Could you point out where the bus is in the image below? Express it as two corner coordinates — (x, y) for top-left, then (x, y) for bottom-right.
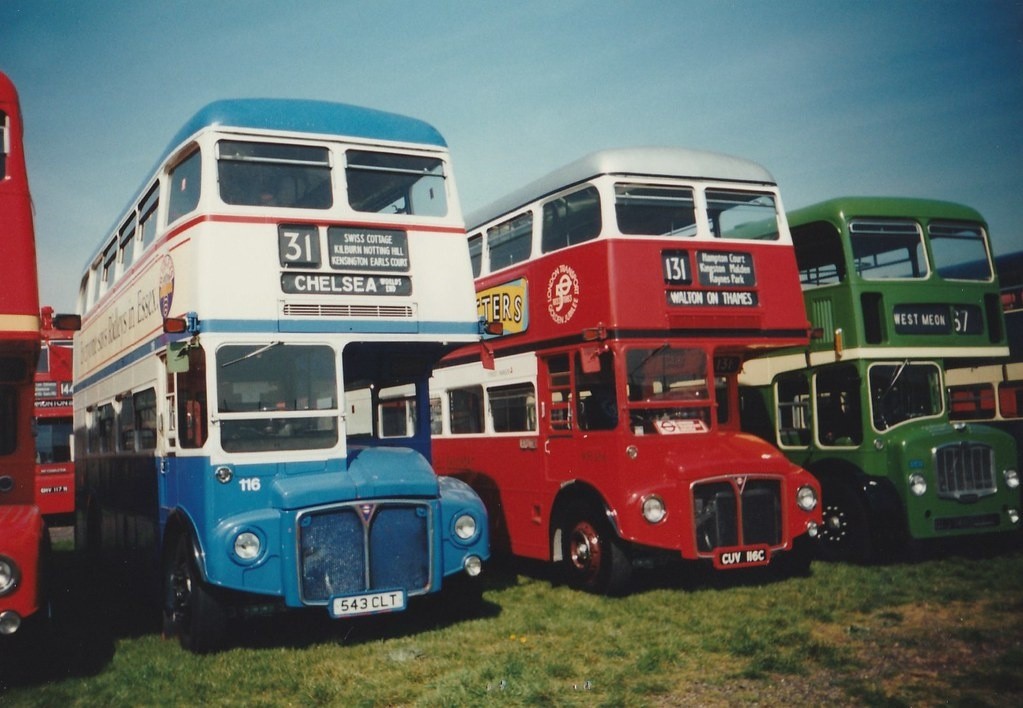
(76, 97), (494, 618)
(1, 72), (204, 637)
(525, 195), (1023, 542)
(278, 148), (824, 594)
